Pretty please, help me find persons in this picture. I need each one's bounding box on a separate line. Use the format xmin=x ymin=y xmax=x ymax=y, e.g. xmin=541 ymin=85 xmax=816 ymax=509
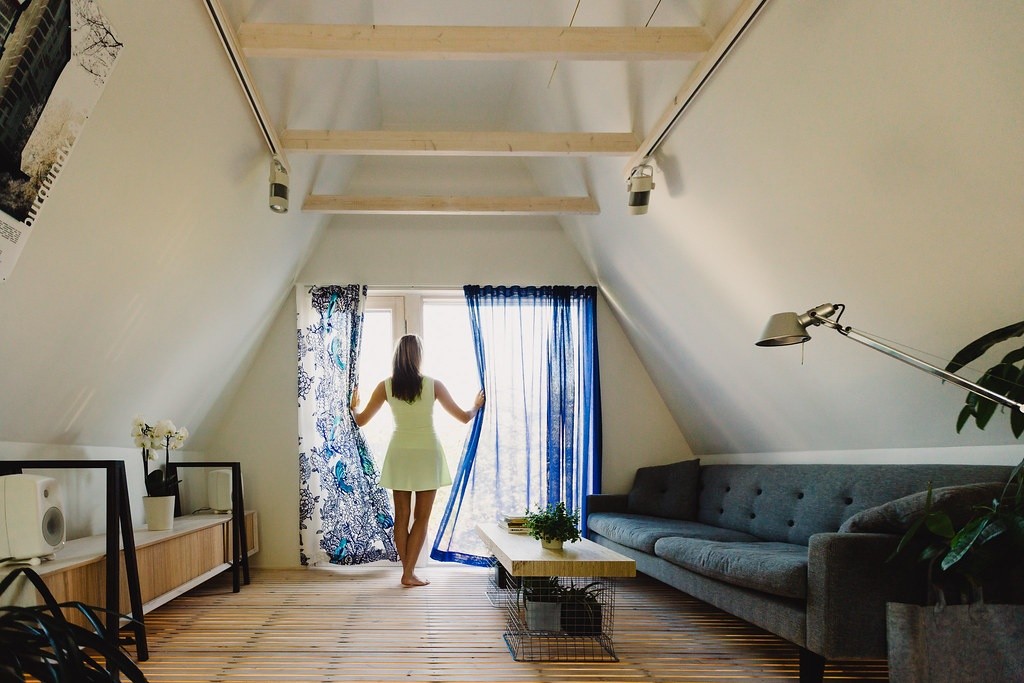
xmin=351 ymin=335 xmax=485 ymax=586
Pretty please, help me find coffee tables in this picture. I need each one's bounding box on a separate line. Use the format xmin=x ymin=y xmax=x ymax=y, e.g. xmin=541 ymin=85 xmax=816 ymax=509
xmin=476 ymin=523 xmax=636 ymax=663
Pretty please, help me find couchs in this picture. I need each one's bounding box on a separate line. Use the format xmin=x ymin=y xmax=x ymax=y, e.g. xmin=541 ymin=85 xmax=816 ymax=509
xmin=584 ymin=463 xmax=1024 ymax=683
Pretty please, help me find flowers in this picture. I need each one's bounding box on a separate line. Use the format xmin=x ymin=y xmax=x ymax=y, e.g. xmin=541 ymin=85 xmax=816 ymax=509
xmin=131 ymin=415 xmax=188 ymax=497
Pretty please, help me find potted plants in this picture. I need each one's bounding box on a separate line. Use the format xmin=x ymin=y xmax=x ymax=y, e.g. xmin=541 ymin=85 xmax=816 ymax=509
xmin=517 ymin=575 xmax=572 ymax=632
xmin=521 ymin=500 xmax=582 ymax=549
xmin=562 ymin=579 xmax=609 ymax=636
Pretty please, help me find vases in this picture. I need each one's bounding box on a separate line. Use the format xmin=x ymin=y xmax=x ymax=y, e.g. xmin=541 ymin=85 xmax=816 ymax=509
xmin=142 ymin=496 xmax=175 ymax=532
xmin=495 ymin=563 xmax=522 ymax=589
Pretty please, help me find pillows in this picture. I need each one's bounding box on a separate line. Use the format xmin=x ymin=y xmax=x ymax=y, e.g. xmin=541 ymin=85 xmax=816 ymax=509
xmin=624 ymin=458 xmax=701 ymax=522
xmin=836 ymin=482 xmax=1018 ymax=534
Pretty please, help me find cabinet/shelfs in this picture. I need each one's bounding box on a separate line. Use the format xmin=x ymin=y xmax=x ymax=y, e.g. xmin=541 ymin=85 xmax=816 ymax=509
xmin=0 ymin=460 xmax=260 ymax=664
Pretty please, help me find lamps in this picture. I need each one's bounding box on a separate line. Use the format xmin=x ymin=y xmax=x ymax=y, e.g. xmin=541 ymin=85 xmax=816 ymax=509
xmin=269 ymin=154 xmax=289 ymax=213
xmin=627 ymin=165 xmax=655 ymax=215
xmin=755 ymin=303 xmax=1024 ymax=414
